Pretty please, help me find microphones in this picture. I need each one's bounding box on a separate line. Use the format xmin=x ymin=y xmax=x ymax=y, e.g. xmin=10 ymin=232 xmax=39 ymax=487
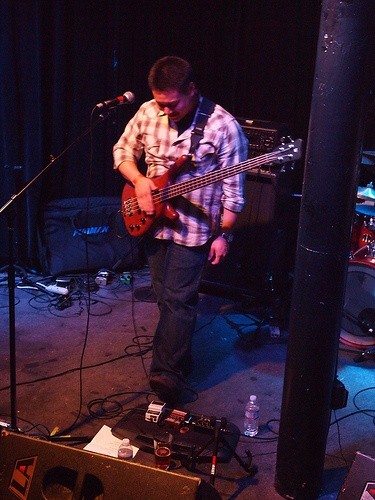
xmin=96 ymin=91 xmax=134 ymax=109
xmin=360 ymin=320 xmax=375 ymax=333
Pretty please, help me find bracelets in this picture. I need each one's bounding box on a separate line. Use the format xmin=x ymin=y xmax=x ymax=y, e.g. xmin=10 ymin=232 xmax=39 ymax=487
xmin=133 ymin=174 xmax=143 ymax=186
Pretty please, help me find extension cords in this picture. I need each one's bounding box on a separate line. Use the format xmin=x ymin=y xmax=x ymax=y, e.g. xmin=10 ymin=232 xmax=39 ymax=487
xmin=46 ymin=284 xmax=69 ymax=295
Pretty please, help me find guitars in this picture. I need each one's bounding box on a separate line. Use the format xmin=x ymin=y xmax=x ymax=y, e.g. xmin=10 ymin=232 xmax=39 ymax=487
xmin=120 ymin=131 xmax=303 ymax=236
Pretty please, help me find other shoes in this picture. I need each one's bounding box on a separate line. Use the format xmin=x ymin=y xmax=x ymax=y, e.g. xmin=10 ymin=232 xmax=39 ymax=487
xmin=149 ymin=373 xmax=182 ymax=403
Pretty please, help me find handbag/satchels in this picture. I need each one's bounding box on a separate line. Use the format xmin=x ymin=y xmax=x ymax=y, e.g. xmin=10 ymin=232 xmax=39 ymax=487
xmin=34 ymin=195 xmax=145 ymax=279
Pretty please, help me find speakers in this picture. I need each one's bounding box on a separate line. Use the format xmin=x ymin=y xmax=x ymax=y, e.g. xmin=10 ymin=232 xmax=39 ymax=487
xmin=197 ymin=172 xmax=302 ymax=312
xmin=0 ymin=427 xmax=224 ymax=500
xmin=338 ymin=451 xmax=375 ymax=500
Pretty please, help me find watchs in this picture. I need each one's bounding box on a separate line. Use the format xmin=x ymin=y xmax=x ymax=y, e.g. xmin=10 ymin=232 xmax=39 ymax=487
xmin=220 ymin=232 xmax=233 ymax=242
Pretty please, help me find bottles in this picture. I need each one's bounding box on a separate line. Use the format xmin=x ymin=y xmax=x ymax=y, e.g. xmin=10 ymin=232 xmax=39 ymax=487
xmin=243 ymin=395 xmax=261 ymax=437
xmin=117 ymin=438 xmax=134 ymax=461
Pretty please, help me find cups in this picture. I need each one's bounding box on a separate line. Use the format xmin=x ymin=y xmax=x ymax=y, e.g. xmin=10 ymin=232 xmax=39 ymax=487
xmin=153 ymin=432 xmax=174 ymax=471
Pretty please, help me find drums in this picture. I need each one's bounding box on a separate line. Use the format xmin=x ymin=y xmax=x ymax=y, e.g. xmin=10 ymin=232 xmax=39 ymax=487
xmin=350 ymin=203 xmax=375 ymax=257
xmin=338 ymin=250 xmax=375 ymax=353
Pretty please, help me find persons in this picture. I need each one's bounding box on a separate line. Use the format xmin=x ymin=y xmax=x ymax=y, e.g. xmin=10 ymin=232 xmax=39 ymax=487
xmin=113 ymin=56 xmax=248 ymax=400
